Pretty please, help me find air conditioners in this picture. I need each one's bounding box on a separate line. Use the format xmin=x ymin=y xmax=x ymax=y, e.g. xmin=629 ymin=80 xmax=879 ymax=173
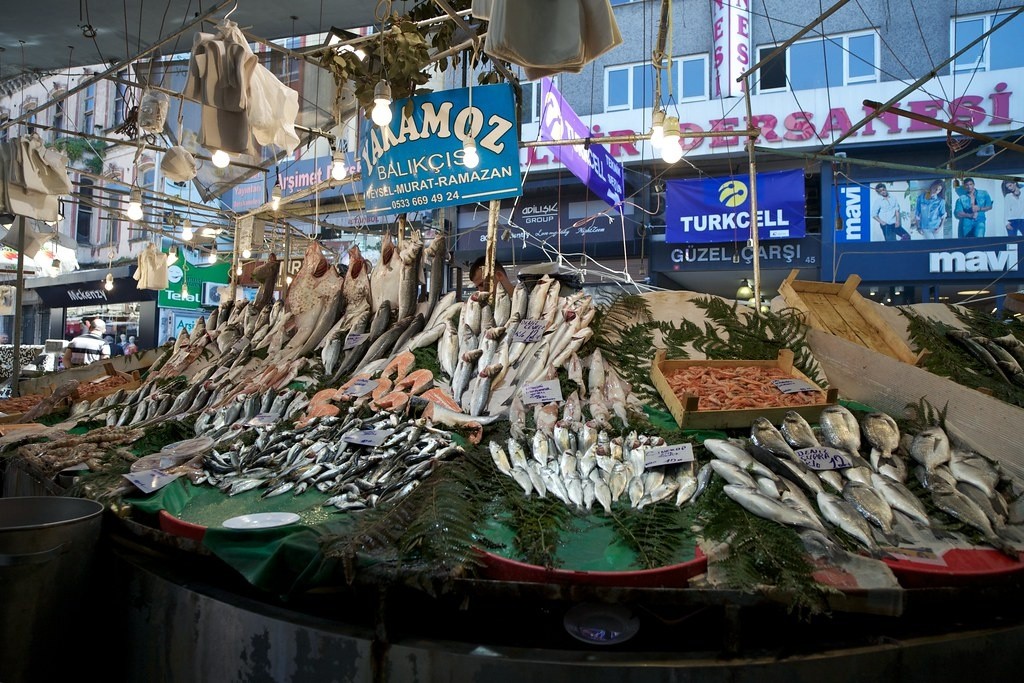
xmin=200 ymin=281 xmax=229 ymax=307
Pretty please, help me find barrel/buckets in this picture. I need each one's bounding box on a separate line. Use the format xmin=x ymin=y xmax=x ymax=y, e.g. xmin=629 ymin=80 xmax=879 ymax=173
xmin=0 ymin=496 xmax=105 ymax=683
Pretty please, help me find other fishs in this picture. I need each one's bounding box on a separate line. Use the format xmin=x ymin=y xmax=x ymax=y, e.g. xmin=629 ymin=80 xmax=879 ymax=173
xmin=490 ymin=418 xmax=712 ymax=516
xmin=992 ymin=331 xmax=1024 ymax=367
xmin=67 ymin=231 xmax=448 ymax=416
xmin=191 ymin=415 xmax=463 ymax=511
xmin=945 ymin=329 xmax=1012 ymax=387
xmin=703 ymin=404 xmax=1024 ymax=561
xmin=973 ymin=336 xmax=1024 ymax=386
xmin=437 ymin=273 xmax=596 ymax=426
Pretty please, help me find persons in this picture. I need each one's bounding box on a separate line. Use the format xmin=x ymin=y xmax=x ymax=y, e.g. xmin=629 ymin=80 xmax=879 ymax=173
xmin=954 ymin=178 xmax=992 ymax=238
xmin=915 ymin=180 xmax=947 ymax=239
xmin=63 ymin=319 xmax=110 ymax=368
xmin=0 ymin=333 xmax=8 ymax=344
xmin=1003 ymin=181 xmax=1024 ymax=236
xmin=469 ymin=256 xmax=514 ymax=299
xmin=125 ymin=336 xmax=138 ymax=355
xmin=872 ymin=184 xmax=911 ymax=240
xmin=104 ymin=336 xmax=124 ymax=357
xmin=118 ymin=334 xmax=127 ymax=350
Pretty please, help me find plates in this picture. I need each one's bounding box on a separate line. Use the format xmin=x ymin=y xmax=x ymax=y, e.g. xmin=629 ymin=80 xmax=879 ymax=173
xmin=221 ymin=512 xmax=301 ymax=529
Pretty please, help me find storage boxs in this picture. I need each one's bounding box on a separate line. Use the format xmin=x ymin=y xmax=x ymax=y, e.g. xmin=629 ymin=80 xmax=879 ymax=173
xmin=651 ymin=350 xmax=839 ymax=427
xmin=777 ymin=269 xmax=934 ymax=369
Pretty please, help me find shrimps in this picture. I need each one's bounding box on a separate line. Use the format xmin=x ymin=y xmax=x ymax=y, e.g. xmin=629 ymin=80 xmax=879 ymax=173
xmin=19 ymin=422 xmax=144 ymax=475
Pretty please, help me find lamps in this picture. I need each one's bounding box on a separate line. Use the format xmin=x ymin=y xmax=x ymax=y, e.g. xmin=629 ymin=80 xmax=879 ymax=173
xmin=735 ymin=278 xmax=754 ymax=301
